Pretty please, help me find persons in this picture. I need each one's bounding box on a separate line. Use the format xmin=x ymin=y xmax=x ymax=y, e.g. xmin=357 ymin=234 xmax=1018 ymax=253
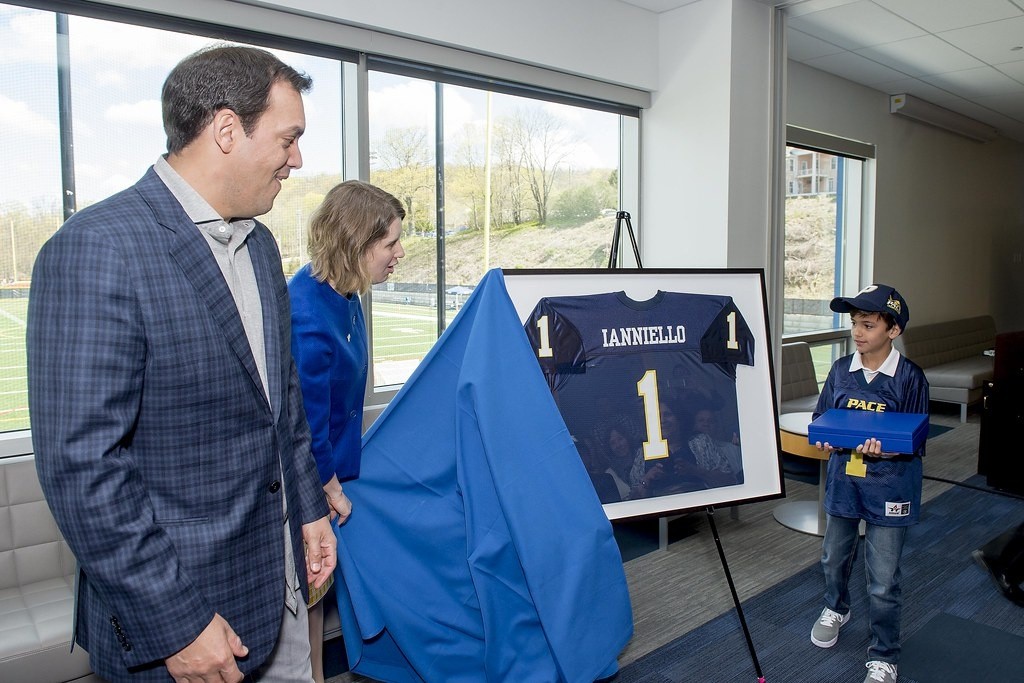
xmin=569 ymin=400 xmax=745 ymax=505
xmin=808 ymin=284 xmax=929 ymax=683
xmin=25 ymin=48 xmax=337 ymax=683
xmin=285 ymin=179 xmax=406 ymax=610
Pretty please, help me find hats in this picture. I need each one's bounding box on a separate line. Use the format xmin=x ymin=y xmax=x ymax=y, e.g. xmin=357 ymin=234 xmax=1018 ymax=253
xmin=830 ymin=284 xmax=909 ymax=336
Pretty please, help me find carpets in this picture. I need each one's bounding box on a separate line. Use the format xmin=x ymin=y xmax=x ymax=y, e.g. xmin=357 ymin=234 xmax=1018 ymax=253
xmin=603 ymin=472 xmax=1024 ymax=683
xmin=927 ymin=423 xmax=955 ymax=439
xmin=611 ymin=520 xmax=700 ymax=565
xmin=781 ymin=452 xmax=820 ymax=486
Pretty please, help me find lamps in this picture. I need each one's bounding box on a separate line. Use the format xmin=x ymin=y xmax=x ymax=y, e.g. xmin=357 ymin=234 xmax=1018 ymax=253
xmin=890 ymin=93 xmax=1000 ymax=143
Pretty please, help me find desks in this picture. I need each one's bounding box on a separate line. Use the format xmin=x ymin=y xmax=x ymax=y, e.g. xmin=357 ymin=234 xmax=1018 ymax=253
xmin=771 ymin=410 xmax=866 ymax=538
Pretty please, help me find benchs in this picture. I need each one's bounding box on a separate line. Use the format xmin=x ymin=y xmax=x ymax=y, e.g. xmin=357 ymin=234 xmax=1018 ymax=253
xmin=891 ymin=313 xmax=998 ymax=424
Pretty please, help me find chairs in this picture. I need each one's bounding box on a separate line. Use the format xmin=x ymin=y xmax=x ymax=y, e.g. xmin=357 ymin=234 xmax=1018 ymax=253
xmin=781 ymin=341 xmax=827 ymax=413
xmin=0 ymin=452 xmax=101 ymax=683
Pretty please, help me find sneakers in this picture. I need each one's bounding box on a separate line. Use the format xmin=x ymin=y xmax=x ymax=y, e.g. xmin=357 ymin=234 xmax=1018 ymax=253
xmin=811 ymin=605 xmax=851 ymax=649
xmin=863 ymin=660 xmax=898 ymax=683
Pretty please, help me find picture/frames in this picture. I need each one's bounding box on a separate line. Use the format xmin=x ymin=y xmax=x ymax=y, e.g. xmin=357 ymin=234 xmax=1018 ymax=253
xmin=499 ymin=266 xmax=788 ymax=522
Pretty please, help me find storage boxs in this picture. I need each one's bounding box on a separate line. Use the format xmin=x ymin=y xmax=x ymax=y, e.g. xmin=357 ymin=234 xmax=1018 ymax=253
xmin=808 ymin=408 xmax=933 ymax=456
